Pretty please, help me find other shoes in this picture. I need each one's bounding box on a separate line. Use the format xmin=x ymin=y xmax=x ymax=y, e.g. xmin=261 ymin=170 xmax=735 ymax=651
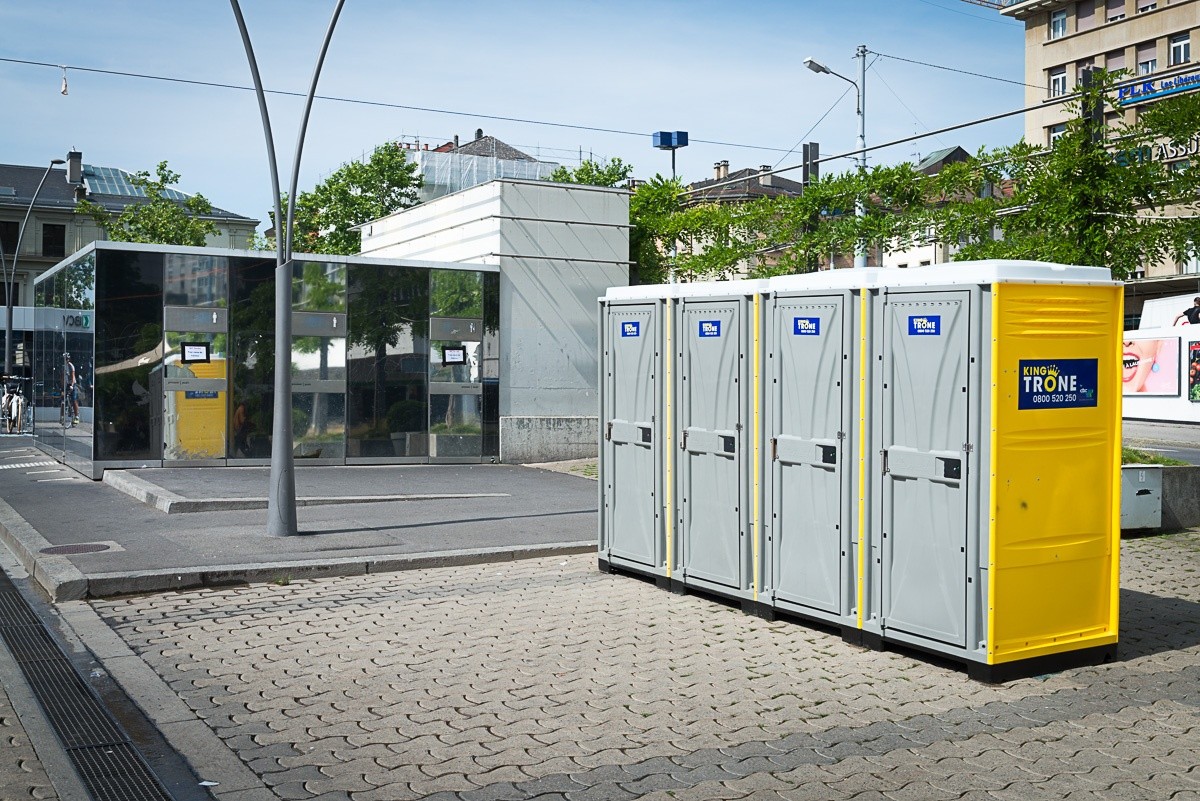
xmin=73 ymin=417 xmax=79 ymax=423
xmin=60 ymin=416 xmax=64 ymax=424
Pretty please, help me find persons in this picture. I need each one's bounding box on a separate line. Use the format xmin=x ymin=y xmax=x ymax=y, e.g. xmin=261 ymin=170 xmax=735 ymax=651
xmin=1122 ymin=339 xmax=1162 ymax=393
xmin=1173 ymin=297 xmax=1200 ymax=326
xmin=235 ymin=399 xmax=251 ymax=457
xmin=57 ymin=352 xmax=80 ymax=423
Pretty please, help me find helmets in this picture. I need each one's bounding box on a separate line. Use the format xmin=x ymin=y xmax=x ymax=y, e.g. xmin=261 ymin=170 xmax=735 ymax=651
xmin=63 ymin=352 xmax=70 ymax=358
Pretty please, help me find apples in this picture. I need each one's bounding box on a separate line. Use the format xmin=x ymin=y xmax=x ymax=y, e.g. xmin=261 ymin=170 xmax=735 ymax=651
xmin=1190 ymin=362 xmax=1200 ymax=384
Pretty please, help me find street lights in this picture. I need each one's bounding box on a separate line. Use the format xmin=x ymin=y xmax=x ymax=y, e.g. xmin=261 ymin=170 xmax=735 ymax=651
xmin=802 ymin=56 xmax=869 ymax=267
xmin=1 ymin=158 xmax=67 ymax=374
xmin=652 ymin=131 xmax=691 ymax=286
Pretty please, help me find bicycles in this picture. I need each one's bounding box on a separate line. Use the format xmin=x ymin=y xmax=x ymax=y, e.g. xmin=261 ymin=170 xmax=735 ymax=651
xmin=60 ymin=388 xmax=79 ymax=429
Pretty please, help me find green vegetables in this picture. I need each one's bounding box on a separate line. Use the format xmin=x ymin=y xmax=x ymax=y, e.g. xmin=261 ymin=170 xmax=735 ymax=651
xmin=1189 ymin=382 xmax=1200 ymax=400
